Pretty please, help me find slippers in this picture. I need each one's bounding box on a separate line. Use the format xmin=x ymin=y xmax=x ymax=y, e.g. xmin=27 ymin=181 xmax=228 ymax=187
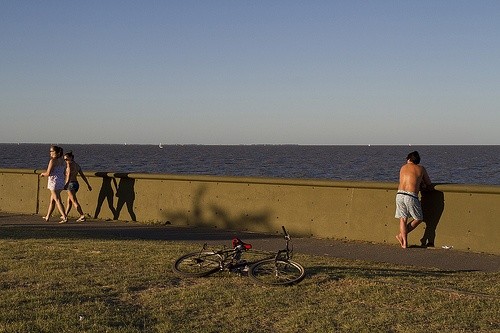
xmin=60 ymin=216 xmax=63 ymax=219
xmin=77 ymin=218 xmax=86 ymax=222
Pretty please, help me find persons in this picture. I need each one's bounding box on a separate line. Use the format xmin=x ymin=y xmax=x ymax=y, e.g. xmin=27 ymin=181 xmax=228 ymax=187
xmin=59 ymin=152 xmax=92 ymax=223
xmin=394 ymin=151 xmax=432 ymax=250
xmin=38 ymin=146 xmax=68 ymax=224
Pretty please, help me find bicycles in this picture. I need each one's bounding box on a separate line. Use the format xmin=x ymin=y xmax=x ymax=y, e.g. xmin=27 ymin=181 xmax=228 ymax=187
xmin=173 ymin=225 xmax=306 ymax=288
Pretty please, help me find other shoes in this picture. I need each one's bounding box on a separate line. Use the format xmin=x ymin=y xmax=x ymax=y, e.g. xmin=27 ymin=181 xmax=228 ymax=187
xmin=59 ymin=220 xmax=68 ymax=223
xmin=42 ymin=216 xmax=48 ymax=221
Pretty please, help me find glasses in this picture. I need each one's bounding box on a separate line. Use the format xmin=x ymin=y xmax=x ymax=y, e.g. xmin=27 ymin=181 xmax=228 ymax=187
xmin=64 ymin=159 xmax=69 ymax=161
